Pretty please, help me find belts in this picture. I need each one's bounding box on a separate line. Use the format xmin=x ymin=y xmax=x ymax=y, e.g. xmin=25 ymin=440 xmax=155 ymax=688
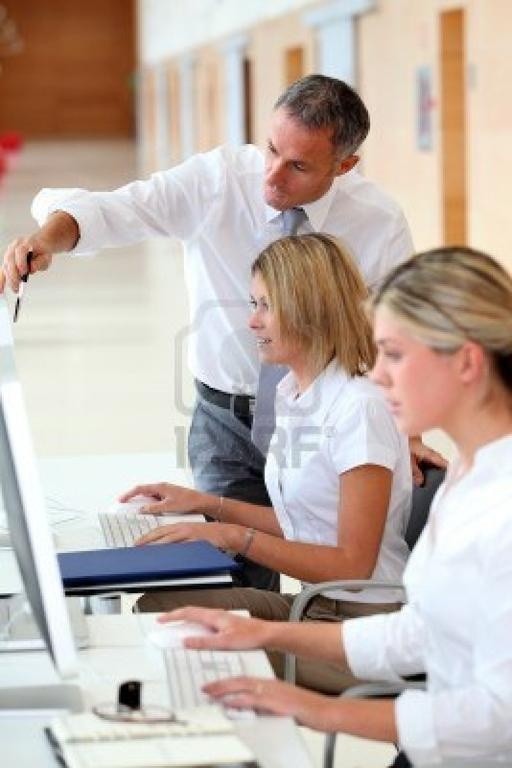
xmin=194 ymin=378 xmax=256 ymax=416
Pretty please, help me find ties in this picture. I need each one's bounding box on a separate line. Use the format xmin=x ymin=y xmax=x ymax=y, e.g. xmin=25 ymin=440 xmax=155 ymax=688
xmin=282 ymin=207 xmax=305 ymax=235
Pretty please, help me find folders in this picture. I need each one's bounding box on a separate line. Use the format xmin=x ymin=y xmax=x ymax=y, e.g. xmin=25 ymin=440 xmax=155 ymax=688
xmin=57 ymin=541 xmax=239 ymax=594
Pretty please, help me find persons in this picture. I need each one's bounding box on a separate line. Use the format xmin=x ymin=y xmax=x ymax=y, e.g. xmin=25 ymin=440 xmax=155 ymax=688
xmin=116 ymin=231 xmax=409 ymax=694
xmin=0 ymin=73 xmax=451 ymax=596
xmin=157 ymin=247 xmax=512 ymax=767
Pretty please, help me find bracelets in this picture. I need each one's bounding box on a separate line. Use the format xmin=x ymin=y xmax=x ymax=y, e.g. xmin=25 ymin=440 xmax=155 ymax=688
xmin=214 ymin=495 xmax=223 ymax=522
xmin=241 ymin=529 xmax=256 ymax=558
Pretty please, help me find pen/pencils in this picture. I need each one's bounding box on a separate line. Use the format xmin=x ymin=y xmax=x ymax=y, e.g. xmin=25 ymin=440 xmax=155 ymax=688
xmin=13 ymin=245 xmax=34 ymax=322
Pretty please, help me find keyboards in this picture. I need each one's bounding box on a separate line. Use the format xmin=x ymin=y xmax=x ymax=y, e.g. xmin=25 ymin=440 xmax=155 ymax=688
xmin=99 ymin=508 xmax=160 ymax=548
xmin=162 ymin=643 xmax=256 ymax=722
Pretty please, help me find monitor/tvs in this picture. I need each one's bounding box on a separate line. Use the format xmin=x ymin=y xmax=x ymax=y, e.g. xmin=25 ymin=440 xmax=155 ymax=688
xmin=0 ymin=297 xmax=94 ymax=680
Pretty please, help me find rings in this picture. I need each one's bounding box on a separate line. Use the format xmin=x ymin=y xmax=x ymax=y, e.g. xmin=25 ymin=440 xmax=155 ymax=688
xmin=258 ymin=684 xmax=266 ymax=695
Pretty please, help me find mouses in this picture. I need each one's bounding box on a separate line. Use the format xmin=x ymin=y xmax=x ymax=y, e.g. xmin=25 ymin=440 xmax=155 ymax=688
xmin=148 ymin=622 xmax=215 ymax=647
xmin=116 ymin=495 xmax=159 ymax=513
